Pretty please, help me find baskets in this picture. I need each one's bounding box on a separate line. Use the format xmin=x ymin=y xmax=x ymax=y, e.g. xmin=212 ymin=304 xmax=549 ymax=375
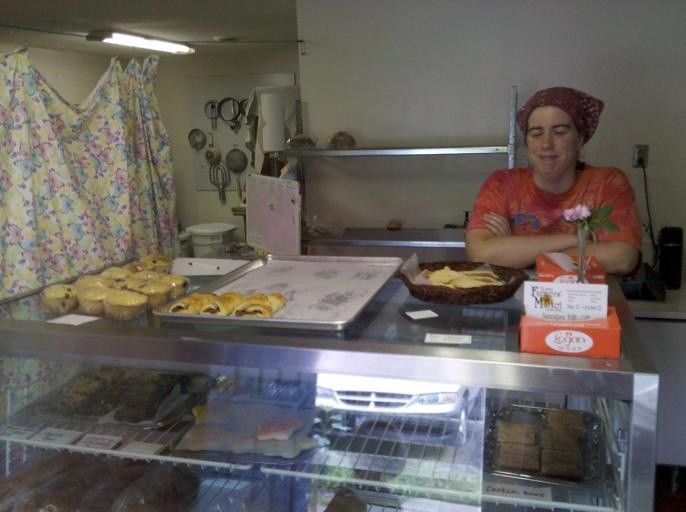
xmin=397 ymin=261 xmax=530 ymax=305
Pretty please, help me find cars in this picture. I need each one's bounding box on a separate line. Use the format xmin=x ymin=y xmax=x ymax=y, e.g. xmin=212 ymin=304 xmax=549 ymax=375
xmin=312 ymin=376 xmax=483 ymax=446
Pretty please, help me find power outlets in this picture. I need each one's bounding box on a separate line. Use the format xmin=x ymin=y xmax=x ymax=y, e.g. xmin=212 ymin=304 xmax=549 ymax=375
xmin=632 ymin=145 xmax=649 ymax=168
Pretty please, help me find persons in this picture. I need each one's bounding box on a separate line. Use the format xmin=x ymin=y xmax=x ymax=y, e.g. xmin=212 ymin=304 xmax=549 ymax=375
xmin=465 ymin=88 xmax=643 ymax=278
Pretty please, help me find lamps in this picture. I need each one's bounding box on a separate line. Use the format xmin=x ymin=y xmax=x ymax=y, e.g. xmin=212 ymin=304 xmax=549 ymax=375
xmin=87 ymin=29 xmax=197 ymax=54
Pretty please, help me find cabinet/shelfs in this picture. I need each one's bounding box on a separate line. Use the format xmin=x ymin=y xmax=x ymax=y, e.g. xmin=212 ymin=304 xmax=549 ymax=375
xmin=267 ymin=84 xmax=519 ymax=255
xmin=0 ymin=253 xmax=660 ymax=512
xmin=633 ymin=320 xmax=685 ymax=496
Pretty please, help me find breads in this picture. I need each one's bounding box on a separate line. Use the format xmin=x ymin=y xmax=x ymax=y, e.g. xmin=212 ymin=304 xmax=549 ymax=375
xmin=418 ymin=264 xmax=505 ymax=288
xmin=40 ymin=254 xmax=287 ymax=321
xmin=495 ymin=410 xmax=586 ymax=480
xmin=0 ymin=371 xmax=366 ymax=512
